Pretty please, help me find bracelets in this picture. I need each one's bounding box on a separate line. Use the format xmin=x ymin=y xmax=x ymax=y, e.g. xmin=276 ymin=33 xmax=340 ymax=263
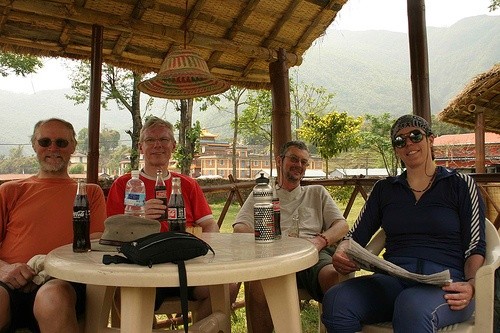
xmin=315 ymin=233 xmax=328 ymax=248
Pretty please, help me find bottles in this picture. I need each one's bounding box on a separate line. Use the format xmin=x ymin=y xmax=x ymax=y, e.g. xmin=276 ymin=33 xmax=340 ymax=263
xmin=155 ymin=169 xmax=168 ymax=221
xmin=287 ymin=214 xmax=300 ymax=240
xmin=72 ymin=179 xmax=91 ymax=253
xmin=252 ymin=172 xmax=275 ymax=243
xmin=123 ymin=170 xmax=147 ymax=219
xmin=271 ymin=197 xmax=282 ymax=240
xmin=166 ymin=177 xmax=187 ymax=234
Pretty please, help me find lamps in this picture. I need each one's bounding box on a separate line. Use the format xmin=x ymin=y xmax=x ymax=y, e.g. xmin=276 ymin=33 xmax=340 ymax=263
xmin=137 ymin=0 xmax=230 ymax=100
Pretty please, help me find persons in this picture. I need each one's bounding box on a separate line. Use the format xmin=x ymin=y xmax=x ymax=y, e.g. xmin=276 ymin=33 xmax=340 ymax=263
xmin=227 ymin=142 xmax=351 ymax=333
xmin=0 ymin=119 xmax=107 ymax=332
xmin=319 ymin=115 xmax=486 ymax=333
xmin=107 ymin=118 xmax=236 ymax=330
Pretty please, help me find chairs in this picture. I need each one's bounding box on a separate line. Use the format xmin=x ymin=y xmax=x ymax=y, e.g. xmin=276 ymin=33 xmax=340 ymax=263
xmin=355 ymin=213 xmax=500 ymax=333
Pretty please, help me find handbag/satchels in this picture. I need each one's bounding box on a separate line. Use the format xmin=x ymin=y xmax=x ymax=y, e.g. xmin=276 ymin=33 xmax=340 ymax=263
xmin=120 ymin=230 xmax=208 ymax=267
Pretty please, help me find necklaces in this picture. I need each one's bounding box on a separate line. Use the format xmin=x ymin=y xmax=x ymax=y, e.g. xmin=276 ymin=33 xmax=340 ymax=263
xmin=405 ymin=166 xmax=437 ymax=193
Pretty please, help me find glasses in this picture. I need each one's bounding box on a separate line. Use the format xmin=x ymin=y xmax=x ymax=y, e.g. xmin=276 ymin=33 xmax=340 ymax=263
xmin=282 ymin=155 xmax=307 ymax=167
xmin=393 ymin=130 xmax=426 ymax=148
xmin=35 ymin=136 xmax=73 ymax=148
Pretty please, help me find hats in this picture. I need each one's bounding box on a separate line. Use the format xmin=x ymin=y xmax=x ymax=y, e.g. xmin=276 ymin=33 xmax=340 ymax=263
xmin=91 ymin=214 xmax=161 ymax=252
xmin=391 ymin=115 xmax=431 ymax=138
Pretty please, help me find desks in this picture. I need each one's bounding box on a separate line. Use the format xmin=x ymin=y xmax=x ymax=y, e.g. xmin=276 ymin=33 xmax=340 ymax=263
xmin=44 ymin=232 xmax=319 ymax=333
